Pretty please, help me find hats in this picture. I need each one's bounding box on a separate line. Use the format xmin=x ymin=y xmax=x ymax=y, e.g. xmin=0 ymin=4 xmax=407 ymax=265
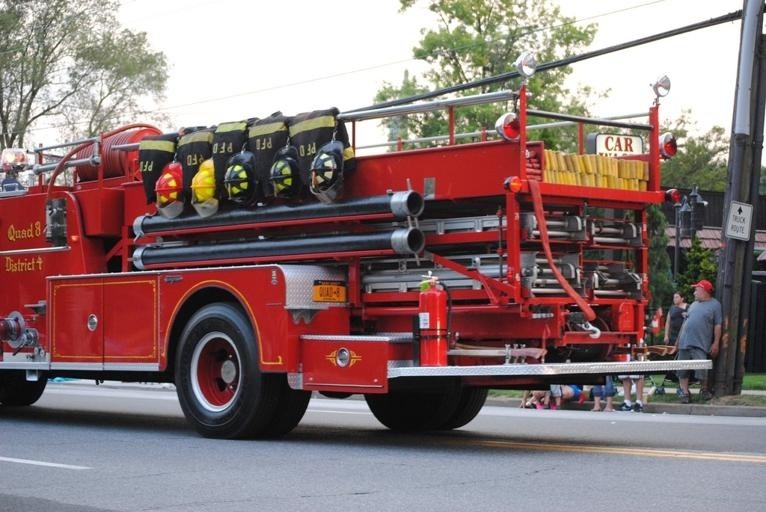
xmin=691 ymin=280 xmax=713 ymax=293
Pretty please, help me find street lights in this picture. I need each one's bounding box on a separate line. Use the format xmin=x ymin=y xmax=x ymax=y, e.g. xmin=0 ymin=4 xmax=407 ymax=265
xmin=673 ymin=182 xmax=708 ymax=280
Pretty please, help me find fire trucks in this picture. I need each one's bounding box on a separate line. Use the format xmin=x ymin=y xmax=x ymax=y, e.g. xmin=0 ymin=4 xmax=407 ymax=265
xmin=0 ymin=51 xmax=713 ymax=436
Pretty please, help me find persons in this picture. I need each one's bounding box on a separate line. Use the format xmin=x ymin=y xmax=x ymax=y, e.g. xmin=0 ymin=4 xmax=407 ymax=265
xmin=520 ymin=338 xmax=646 ymax=412
xmin=664 ymin=291 xmax=690 ymax=346
xmin=677 ymin=280 xmax=723 ymax=404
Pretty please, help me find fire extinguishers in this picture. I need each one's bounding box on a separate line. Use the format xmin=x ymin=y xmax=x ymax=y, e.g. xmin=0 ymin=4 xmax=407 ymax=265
xmin=419 ymin=270 xmax=452 ymax=367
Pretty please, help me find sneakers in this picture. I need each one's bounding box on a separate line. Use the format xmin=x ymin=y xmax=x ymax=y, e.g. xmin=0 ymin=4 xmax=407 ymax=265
xmin=680 ymin=392 xmax=690 ymax=403
xmin=591 ymin=402 xmax=644 ymax=412
xmin=520 ymin=400 xmax=563 ymax=410
xmin=701 ymin=391 xmax=712 ymax=400
